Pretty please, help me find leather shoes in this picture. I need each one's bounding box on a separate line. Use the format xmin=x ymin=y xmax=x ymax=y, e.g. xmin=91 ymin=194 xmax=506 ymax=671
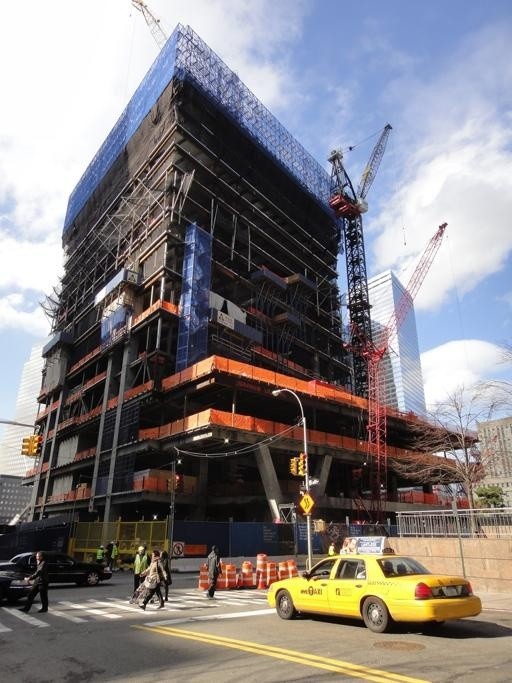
xmin=158 ymin=603 xmax=164 ymax=609
xmin=139 ymin=605 xmax=145 ymax=609
xmin=18 ymin=607 xmax=29 ymax=613
xmin=38 ymin=608 xmax=48 ymax=613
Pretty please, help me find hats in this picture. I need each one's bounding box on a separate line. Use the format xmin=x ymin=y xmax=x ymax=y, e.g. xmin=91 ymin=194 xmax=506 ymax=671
xmin=136 ymin=546 xmax=145 ymax=552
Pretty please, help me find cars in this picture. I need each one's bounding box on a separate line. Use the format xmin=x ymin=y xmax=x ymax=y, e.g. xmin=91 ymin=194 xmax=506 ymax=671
xmin=1 ymin=570 xmax=35 ymax=602
xmin=266 ymin=553 xmax=483 ymax=634
xmin=0 ymin=550 xmax=113 ymax=586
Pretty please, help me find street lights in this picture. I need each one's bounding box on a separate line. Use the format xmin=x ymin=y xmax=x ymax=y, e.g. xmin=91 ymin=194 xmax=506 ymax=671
xmin=271 ymin=387 xmax=313 ymax=569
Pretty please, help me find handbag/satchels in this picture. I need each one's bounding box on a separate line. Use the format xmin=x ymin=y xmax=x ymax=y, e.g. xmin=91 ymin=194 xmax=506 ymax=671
xmin=156 ymin=567 xmax=163 ymax=583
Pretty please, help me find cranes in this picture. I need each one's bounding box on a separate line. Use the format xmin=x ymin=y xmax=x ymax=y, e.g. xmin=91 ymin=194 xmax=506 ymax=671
xmin=326 ymin=122 xmax=393 ymax=399
xmin=342 ymin=221 xmax=449 ymax=502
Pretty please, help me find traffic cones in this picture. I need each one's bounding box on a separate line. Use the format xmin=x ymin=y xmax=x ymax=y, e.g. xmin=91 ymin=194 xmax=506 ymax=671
xmin=215 ymin=553 xmax=298 ymax=591
xmin=197 ymin=563 xmax=210 ymax=592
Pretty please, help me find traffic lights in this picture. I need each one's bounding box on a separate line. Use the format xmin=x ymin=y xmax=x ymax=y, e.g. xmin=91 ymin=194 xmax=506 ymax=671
xmin=21 ymin=436 xmax=32 ymax=456
xmin=289 ymin=457 xmax=297 ymax=476
xmin=300 ymin=452 xmax=307 ymax=470
xmin=31 ymin=436 xmax=42 ymax=456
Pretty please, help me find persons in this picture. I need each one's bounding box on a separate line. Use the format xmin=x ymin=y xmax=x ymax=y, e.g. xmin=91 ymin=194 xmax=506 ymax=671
xmin=207 ymin=545 xmax=222 ymax=598
xmin=328 ymin=543 xmax=336 ymax=556
xmin=18 ymin=552 xmax=48 ymax=612
xmin=139 ymin=552 xmax=167 ymax=611
xmin=155 ymin=551 xmax=172 ymax=601
xmin=134 ymin=545 xmax=151 ymax=591
xmin=96 ymin=539 xmax=119 ymax=572
